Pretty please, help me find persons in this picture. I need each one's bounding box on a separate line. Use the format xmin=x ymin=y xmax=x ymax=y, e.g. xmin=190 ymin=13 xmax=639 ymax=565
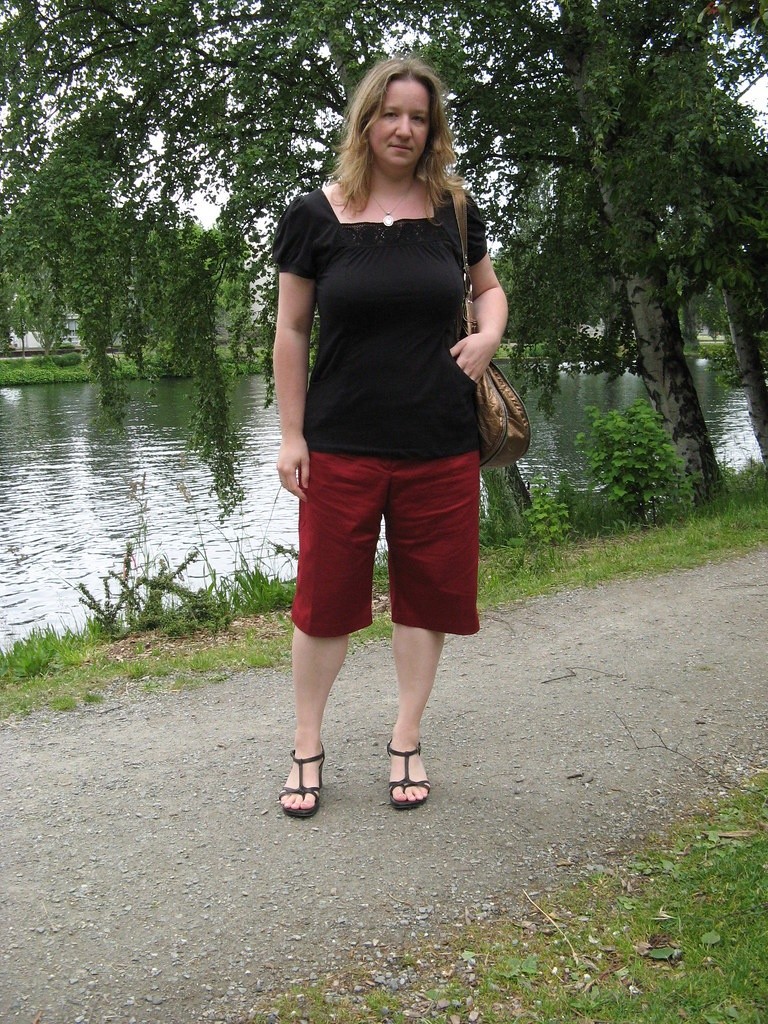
xmin=273 ymin=62 xmax=508 ymax=817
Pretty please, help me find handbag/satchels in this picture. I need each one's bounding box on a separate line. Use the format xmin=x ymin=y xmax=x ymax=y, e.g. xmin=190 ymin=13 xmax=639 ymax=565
xmin=451 ymin=184 xmax=530 ymax=466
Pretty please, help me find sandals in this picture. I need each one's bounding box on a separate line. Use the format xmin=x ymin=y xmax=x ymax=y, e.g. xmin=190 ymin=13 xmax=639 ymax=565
xmin=280 ymin=742 xmax=326 ymax=819
xmin=385 ymin=739 xmax=431 ymax=811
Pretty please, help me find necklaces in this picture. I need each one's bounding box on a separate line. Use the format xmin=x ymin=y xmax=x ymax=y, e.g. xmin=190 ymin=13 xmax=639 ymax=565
xmin=370 ymin=183 xmax=412 ymax=227
